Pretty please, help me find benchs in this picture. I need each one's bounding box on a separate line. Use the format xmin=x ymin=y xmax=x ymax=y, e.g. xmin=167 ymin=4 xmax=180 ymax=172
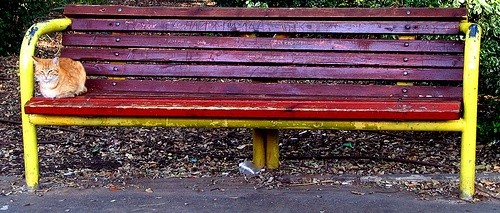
xmin=19 ymin=4 xmax=482 ymax=201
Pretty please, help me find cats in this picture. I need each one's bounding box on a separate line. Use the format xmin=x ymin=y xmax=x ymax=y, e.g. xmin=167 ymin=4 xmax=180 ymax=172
xmin=32 ymin=55 xmax=87 ymax=99
